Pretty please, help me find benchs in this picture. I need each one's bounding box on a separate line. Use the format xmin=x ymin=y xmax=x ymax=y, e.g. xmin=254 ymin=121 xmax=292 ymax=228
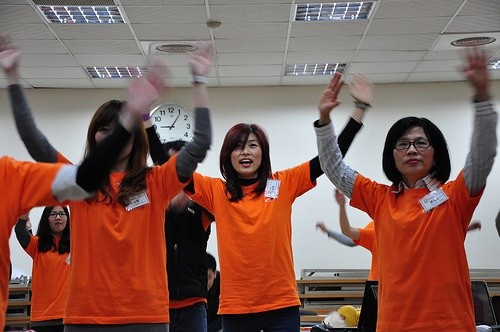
xmin=5 ymin=277 xmax=500 ymax=332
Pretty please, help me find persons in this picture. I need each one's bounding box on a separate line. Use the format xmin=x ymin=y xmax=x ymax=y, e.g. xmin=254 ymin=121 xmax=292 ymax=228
xmin=206 ymin=252 xmax=223 ymax=332
xmin=129 ymin=72 xmax=373 ymax=332
xmin=314 ymin=45 xmax=498 ymax=332
xmin=0 ymin=31 xmax=213 ymax=332
xmin=0 ymin=61 xmax=171 ymax=331
xmin=152 ymin=140 xmax=216 ymax=331
xmin=15 ymin=206 xmax=70 ymax=332
xmin=316 ymin=218 xmax=481 ymax=332
xmin=334 ymin=188 xmax=378 ymax=332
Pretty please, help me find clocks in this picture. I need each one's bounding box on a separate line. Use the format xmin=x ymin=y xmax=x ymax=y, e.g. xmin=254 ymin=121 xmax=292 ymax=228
xmin=149 ymin=103 xmax=195 ymax=144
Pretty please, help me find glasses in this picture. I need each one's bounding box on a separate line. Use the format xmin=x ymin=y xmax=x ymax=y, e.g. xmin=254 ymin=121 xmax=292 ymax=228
xmin=393 ymin=139 xmax=431 ymax=150
xmin=48 ymin=211 xmax=67 ymax=218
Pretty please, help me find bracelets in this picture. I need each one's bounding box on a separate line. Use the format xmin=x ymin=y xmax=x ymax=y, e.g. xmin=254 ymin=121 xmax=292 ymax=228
xmin=353 ymin=100 xmax=368 ymax=110
xmin=191 ymin=74 xmax=209 ymax=84
xmin=142 ymin=113 xmax=151 ymax=122
